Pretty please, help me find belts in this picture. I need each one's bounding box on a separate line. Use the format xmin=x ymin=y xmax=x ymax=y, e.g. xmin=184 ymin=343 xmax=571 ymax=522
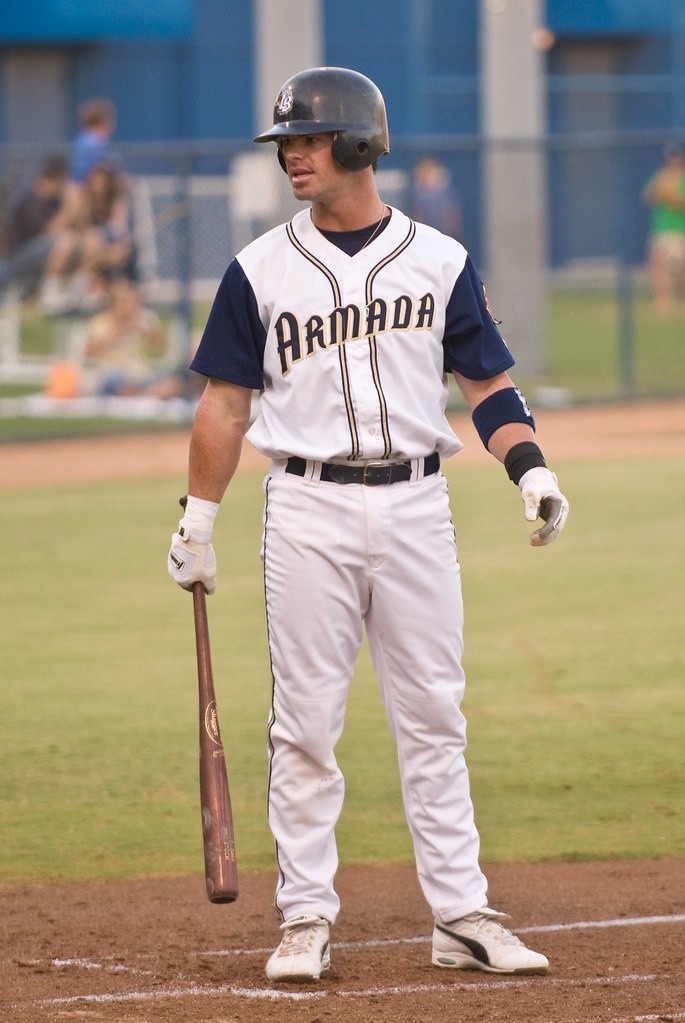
xmin=284 ymin=452 xmax=440 ymax=487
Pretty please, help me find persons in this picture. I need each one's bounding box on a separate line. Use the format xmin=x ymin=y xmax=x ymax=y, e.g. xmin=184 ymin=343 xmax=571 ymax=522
xmin=166 ymin=66 xmax=569 ymax=982
xmin=640 ymin=140 xmax=685 ymax=301
xmin=9 ymin=94 xmax=199 ymax=398
xmin=405 ymin=159 xmax=461 ymax=245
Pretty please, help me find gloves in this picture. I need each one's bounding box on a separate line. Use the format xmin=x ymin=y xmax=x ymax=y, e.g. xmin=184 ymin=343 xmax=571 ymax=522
xmin=167 ymin=495 xmax=220 ymax=595
xmin=517 ymin=466 xmax=568 ymax=546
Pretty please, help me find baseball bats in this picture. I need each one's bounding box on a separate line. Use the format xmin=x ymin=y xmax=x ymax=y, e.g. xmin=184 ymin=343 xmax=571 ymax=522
xmin=178 ymin=494 xmax=241 ymax=907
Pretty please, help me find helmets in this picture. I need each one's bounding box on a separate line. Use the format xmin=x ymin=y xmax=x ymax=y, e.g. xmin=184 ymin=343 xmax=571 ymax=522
xmin=254 ymin=67 xmax=389 ymax=174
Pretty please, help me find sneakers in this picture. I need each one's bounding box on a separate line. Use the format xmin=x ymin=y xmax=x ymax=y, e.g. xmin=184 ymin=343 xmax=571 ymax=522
xmin=430 ymin=907 xmax=550 ymax=973
xmin=264 ymin=915 xmax=330 ymax=982
